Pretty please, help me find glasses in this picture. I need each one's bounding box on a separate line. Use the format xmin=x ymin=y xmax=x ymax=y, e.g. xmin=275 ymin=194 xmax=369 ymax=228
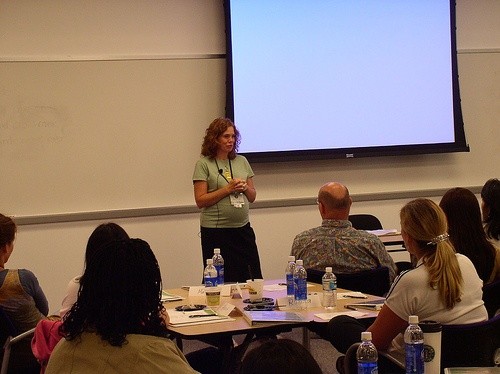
xmin=317 ymin=198 xmax=320 ymax=204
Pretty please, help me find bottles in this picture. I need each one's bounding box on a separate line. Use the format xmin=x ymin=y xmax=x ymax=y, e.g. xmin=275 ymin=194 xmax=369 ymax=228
xmin=405 ymin=315 xmax=424 ymax=374
xmin=203 ymin=259 xmax=218 ymax=286
xmin=294 ymin=260 xmax=307 ymax=310
xmin=212 ymin=248 xmax=224 ymax=290
xmin=322 ymin=266 xmax=337 ymax=311
xmin=356 ymin=331 xmax=378 ymax=374
xmin=287 ymin=255 xmax=296 ymax=306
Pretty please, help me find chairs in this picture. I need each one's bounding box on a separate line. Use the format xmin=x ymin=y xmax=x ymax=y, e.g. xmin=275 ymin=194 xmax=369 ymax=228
xmin=344 ymin=313 xmax=500 ymax=374
xmin=304 ymin=269 xmax=390 ymax=344
xmin=348 ymin=215 xmax=383 ymax=231
xmin=0 ymin=305 xmax=40 ymax=373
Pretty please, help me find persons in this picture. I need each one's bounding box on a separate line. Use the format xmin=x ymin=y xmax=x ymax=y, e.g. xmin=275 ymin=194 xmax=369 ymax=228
xmin=193 ymin=115 xmax=277 ymax=342
xmin=292 ymin=182 xmax=397 ymax=284
xmin=240 ymin=338 xmax=323 ymax=374
xmin=322 ymin=198 xmax=490 ymax=374
xmin=439 ymin=178 xmax=500 ymax=320
xmin=0 ymin=212 xmax=204 ymax=374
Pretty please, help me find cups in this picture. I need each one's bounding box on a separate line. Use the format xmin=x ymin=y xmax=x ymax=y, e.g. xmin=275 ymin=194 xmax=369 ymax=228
xmin=204 ymin=286 xmax=221 ymax=308
xmin=418 ymin=318 xmax=443 ymax=374
xmin=246 ymin=278 xmax=264 ymax=303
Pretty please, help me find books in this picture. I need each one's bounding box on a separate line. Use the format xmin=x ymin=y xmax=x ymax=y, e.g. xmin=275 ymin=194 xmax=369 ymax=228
xmin=344 ymin=298 xmax=386 ymax=311
xmin=365 ymin=229 xmax=402 ymax=236
xmin=241 ymin=311 xmax=303 ymax=326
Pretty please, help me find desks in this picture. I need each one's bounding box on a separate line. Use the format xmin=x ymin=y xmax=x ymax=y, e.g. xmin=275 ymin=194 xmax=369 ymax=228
xmin=378 ymin=230 xmax=417 ymax=268
xmin=160 ymin=278 xmax=385 ymax=374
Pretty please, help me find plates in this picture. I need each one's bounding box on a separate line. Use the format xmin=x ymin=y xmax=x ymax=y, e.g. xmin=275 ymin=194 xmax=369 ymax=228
xmin=244 ymin=298 xmax=274 ymax=304
xmin=245 ymin=304 xmax=275 ymax=311
xmin=175 ymin=304 xmax=206 ymax=311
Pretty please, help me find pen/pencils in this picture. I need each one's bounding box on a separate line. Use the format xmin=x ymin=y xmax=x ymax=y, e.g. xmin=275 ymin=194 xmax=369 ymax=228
xmin=278 ymin=282 xmax=287 ymax=285
xmin=164 ymin=299 xmax=183 ymax=302
xmin=189 ymin=314 xmax=219 ymax=317
xmin=345 ymin=304 xmax=358 ymax=310
xmin=342 ymin=295 xmax=367 ymax=299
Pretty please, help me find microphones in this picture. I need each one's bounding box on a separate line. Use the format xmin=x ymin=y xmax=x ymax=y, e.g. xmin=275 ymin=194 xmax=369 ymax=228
xmin=219 ymin=169 xmax=223 ymax=173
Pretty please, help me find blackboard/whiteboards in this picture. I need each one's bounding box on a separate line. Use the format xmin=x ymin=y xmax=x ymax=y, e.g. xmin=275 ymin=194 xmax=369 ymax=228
xmin=1 ymin=47 xmax=500 ymax=228
